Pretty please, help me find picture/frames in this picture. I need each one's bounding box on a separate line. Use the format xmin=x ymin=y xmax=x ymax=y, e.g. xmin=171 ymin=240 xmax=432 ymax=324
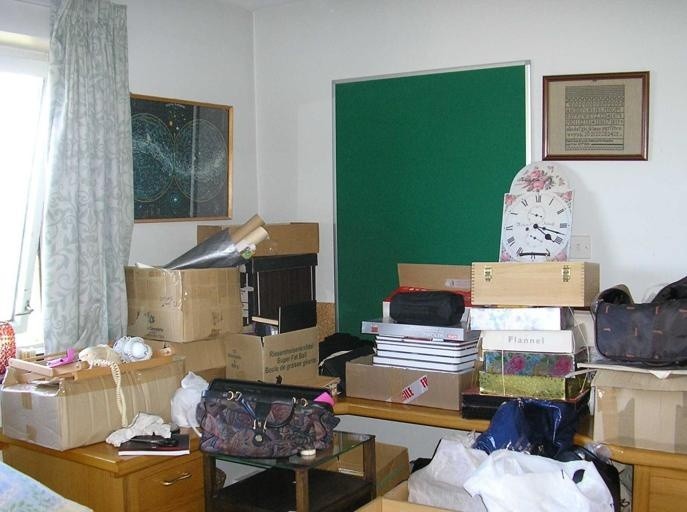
xmin=540 ymin=71 xmax=650 ymax=161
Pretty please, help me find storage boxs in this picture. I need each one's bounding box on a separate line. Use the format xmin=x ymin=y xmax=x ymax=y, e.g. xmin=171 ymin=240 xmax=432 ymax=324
xmin=237 ymin=253 xmax=318 ymax=336
xmin=345 ymin=353 xmax=480 ymax=412
xmin=125 ymin=266 xmax=243 ymax=344
xmin=0 ymin=353 xmax=186 ymax=452
xmin=591 ymin=370 xmax=687 ymax=455
xmin=223 ymin=327 xmax=320 ymax=386
xmin=317 ymin=441 xmax=410 ymax=497
xmin=145 ymin=338 xmax=226 ymax=383
xmin=197 ymin=221 xmax=320 ymax=257
xmin=471 ymin=261 xmax=600 ymax=307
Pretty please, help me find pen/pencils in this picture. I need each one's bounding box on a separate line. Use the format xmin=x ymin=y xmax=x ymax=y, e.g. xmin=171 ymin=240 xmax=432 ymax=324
xmin=129 ymin=438 xmax=177 ymax=449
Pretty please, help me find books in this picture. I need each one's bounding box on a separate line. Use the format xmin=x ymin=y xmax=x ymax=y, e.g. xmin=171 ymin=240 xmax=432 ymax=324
xmin=361 ymin=319 xmax=481 ymax=374
xmin=119 ymin=434 xmax=191 ymax=456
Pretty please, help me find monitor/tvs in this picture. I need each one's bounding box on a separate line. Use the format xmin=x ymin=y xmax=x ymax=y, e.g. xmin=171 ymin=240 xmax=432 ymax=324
xmin=279 ymin=299 xmax=317 ymax=333
xmin=258 ymin=265 xmax=315 ymax=320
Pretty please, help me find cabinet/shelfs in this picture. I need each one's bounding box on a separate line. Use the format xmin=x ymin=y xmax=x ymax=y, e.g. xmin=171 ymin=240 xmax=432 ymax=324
xmin=0 ymin=428 xmax=206 ymax=512
xmin=333 ymin=397 xmax=687 ymax=512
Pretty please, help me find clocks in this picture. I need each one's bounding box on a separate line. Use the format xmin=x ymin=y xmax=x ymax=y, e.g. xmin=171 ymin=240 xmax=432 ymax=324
xmin=499 ymin=162 xmax=575 ymax=263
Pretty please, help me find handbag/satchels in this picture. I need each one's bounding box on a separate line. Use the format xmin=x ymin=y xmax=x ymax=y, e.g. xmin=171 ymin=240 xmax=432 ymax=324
xmin=199 ymin=378 xmax=339 ymax=459
xmin=319 ymin=351 xmax=360 ymax=397
xmin=589 ymin=275 xmax=687 ymax=367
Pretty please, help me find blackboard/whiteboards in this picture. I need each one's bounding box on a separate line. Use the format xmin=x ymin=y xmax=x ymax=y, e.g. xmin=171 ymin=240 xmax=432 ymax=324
xmin=331 ymin=59 xmax=531 ymax=344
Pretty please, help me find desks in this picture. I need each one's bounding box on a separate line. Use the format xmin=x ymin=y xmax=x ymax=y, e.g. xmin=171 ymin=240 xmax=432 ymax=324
xmin=209 ymin=431 xmax=377 ymax=512
